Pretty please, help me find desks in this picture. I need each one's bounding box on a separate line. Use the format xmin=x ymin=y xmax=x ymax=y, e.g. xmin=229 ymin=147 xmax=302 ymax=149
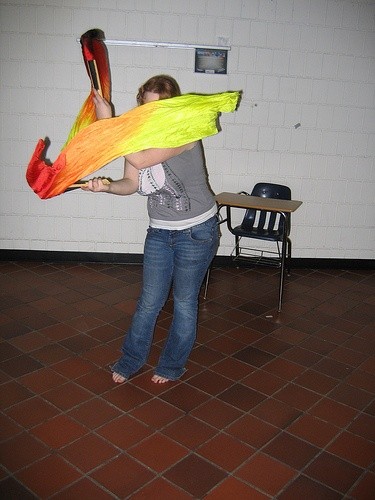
xmin=212 ymin=192 xmax=302 ymax=213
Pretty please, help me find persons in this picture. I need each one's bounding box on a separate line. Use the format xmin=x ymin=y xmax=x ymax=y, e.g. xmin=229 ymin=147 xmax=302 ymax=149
xmin=81 ymin=75 xmax=221 ymax=385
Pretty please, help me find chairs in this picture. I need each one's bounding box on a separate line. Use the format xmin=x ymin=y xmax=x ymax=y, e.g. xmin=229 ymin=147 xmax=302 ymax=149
xmin=204 ymin=183 xmax=303 ymax=312
